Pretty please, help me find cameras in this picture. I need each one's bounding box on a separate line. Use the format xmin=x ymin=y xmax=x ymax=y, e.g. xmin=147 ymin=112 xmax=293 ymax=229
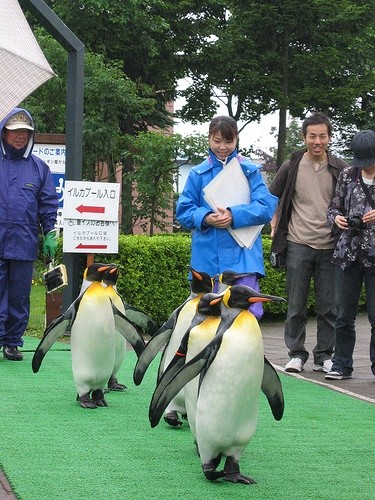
xmin=345 ymin=213 xmax=370 ymax=229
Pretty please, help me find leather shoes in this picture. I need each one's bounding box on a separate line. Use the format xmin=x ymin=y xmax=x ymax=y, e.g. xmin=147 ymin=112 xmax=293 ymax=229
xmin=4 ymin=344 xmax=22 ymax=360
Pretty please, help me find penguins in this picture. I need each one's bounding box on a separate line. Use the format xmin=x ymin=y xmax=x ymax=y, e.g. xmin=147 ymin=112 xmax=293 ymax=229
xmin=149 ymin=285 xmax=288 ymax=485
xmin=149 ymin=292 xmax=225 ymax=458
xmin=133 ymin=266 xmax=215 ymax=428
xmin=216 ymin=270 xmax=255 ymax=295
xmin=32 ymin=263 xmax=146 ymax=409
xmin=43 ymin=265 xmax=158 ymax=389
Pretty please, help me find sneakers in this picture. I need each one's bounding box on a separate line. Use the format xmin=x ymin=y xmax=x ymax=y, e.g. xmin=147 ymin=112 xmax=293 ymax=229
xmin=324 ymin=367 xmax=351 ymax=380
xmin=313 ymin=358 xmax=334 ymax=372
xmin=284 ymin=357 xmax=306 ymax=373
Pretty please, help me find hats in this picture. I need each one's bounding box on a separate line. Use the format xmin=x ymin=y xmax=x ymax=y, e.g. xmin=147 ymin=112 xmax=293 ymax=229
xmin=5 ymin=112 xmax=35 ymax=132
xmin=350 ymin=129 xmax=375 ymax=168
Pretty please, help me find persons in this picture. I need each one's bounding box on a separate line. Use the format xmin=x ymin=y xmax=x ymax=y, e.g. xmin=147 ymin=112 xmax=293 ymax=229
xmin=175 ymin=116 xmax=279 ymax=322
xmin=268 ymin=113 xmax=350 ymax=373
xmin=0 ymin=108 xmax=59 ymax=360
xmin=325 ymin=130 xmax=375 ymax=380
